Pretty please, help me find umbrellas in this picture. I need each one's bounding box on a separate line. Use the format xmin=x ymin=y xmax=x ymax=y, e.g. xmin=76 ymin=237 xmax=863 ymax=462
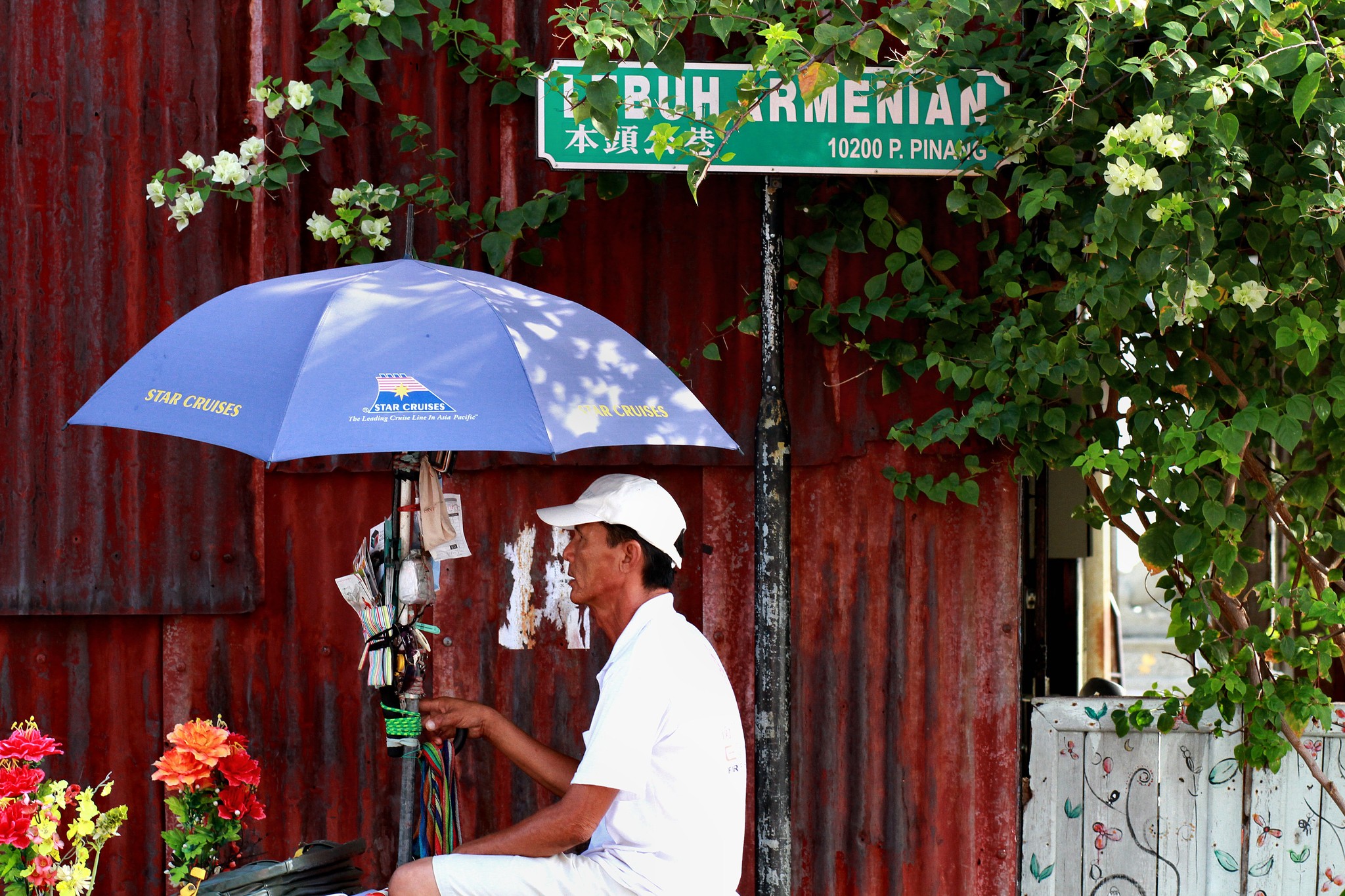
xmin=59 ymin=202 xmax=750 ymax=870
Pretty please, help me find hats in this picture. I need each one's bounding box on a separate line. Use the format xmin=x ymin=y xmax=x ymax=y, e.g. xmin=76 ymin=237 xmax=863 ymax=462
xmin=535 ymin=473 xmax=689 ymax=572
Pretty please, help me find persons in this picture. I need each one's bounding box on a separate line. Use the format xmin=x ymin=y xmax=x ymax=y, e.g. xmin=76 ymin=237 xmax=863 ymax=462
xmin=388 ymin=472 xmax=746 ymax=895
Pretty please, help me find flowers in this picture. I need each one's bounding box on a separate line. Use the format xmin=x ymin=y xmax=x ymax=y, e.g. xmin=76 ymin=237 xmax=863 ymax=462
xmin=144 ymin=711 xmax=272 ymax=896
xmin=0 ymin=711 xmax=132 ymax=896
